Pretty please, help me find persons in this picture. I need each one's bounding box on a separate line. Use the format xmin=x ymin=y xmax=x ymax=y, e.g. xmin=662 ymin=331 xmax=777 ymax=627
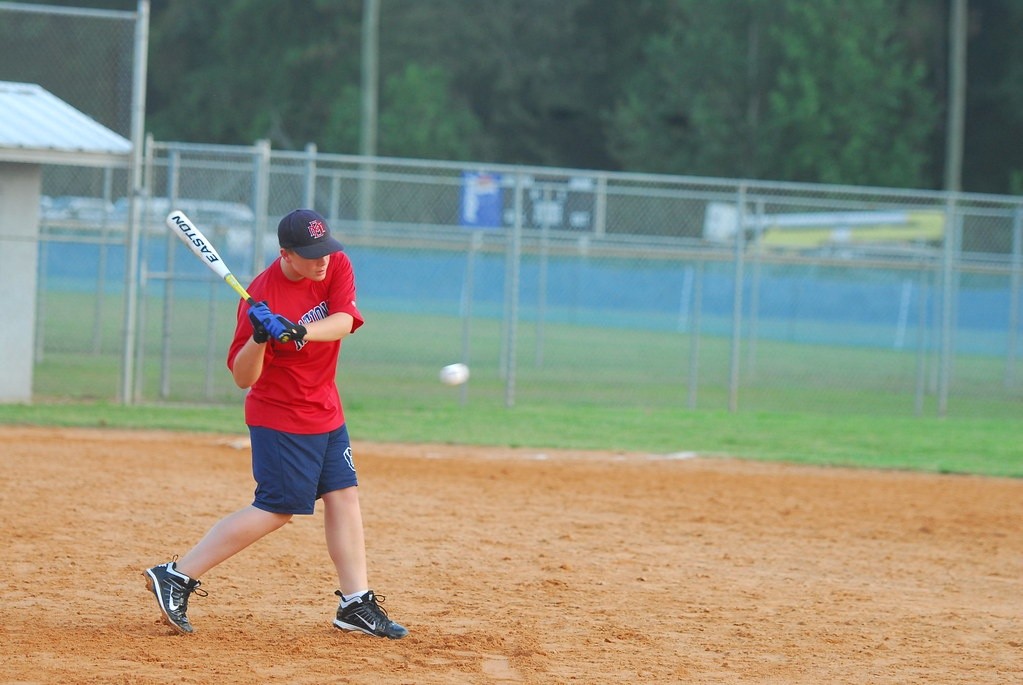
xmin=142 ymin=208 xmax=408 ymax=642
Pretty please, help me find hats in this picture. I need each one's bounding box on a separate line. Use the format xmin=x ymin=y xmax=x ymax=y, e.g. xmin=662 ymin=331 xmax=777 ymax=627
xmin=278 ymin=209 xmax=344 ymax=260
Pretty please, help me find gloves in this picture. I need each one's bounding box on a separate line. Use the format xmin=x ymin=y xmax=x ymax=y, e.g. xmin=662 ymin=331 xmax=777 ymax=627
xmin=246 ymin=300 xmax=273 ymax=344
xmin=262 ymin=314 xmax=307 ymax=343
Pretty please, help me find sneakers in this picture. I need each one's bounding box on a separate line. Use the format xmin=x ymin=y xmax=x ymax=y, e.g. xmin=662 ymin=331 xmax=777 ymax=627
xmin=142 ymin=554 xmax=208 ymax=636
xmin=332 ymin=590 xmax=409 ymax=640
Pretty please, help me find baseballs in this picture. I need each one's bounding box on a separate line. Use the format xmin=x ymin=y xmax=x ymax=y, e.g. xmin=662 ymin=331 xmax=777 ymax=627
xmin=439 ymin=364 xmax=470 ymax=386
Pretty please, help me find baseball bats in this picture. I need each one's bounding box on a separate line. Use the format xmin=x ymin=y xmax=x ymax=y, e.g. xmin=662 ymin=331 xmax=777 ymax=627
xmin=164 ymin=209 xmax=291 ymax=344
